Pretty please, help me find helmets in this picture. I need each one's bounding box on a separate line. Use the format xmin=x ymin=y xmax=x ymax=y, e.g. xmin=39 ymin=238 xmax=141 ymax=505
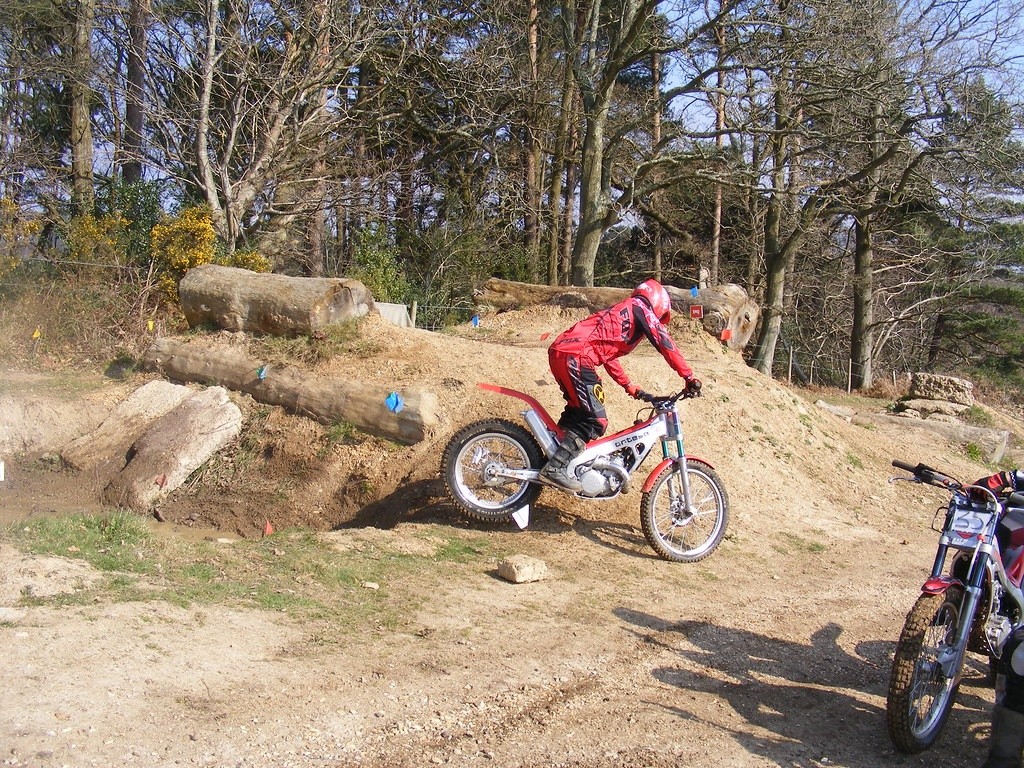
xmin=631 ymin=279 xmax=671 ymax=325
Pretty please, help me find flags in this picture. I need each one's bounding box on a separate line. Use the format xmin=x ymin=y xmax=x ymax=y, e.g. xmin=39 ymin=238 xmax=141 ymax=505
xmin=473 ymin=315 xmax=478 ymax=326
xmin=386 ymin=392 xmax=403 ymax=416
xmin=541 ymin=332 xmax=551 ymax=341
xmin=690 ymin=286 xmax=698 ymax=298
xmin=720 ymin=329 xmax=732 ymax=340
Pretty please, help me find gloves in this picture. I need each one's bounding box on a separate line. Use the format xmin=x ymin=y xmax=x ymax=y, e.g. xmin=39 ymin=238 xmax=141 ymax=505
xmin=684 ymin=374 xmax=702 ymax=393
xmin=969 ymin=471 xmax=1010 ymax=501
xmin=623 ymin=382 xmax=640 ymax=399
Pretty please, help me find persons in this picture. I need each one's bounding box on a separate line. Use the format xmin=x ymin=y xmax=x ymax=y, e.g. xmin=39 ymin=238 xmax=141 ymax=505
xmin=537 ymin=278 xmax=703 ymax=493
xmin=982 ymin=625 xmax=1024 ymax=768
xmin=973 ymin=469 xmax=1024 ymax=497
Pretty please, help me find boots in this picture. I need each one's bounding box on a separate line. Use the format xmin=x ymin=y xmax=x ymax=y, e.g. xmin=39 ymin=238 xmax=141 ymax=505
xmin=538 ymin=431 xmax=586 ymax=493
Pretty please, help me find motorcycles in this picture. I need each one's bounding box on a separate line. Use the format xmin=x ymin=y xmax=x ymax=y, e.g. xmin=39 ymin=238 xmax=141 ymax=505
xmin=882 ymin=457 xmax=1024 ymax=759
xmin=437 ymin=380 xmax=733 ymax=565
